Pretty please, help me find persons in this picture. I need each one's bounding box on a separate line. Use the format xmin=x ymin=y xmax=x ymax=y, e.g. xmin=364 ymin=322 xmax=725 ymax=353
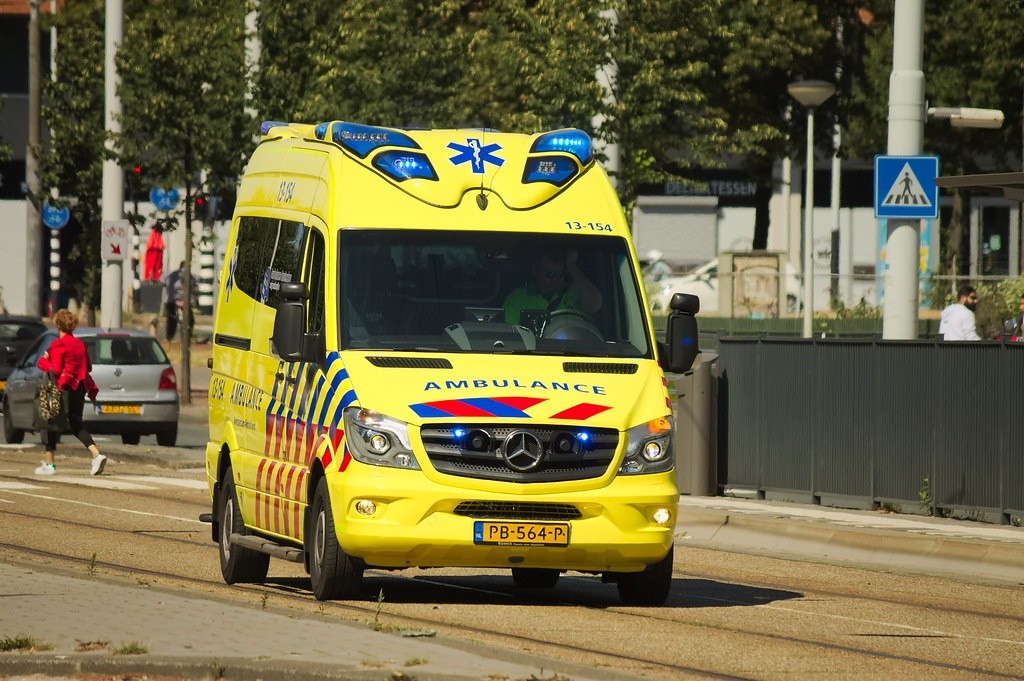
xmin=165 ymin=261 xmax=199 ymax=342
xmin=1019 ymin=293 xmax=1024 ymax=342
xmin=35 ymin=309 xmax=107 ymax=478
xmin=938 ymin=285 xmax=981 ymax=341
xmin=645 ymin=251 xmax=672 ymax=282
xmin=505 ymin=248 xmax=602 ymax=326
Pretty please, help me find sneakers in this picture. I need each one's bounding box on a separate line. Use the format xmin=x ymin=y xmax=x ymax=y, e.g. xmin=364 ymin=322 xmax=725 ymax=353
xmin=91 ymin=455 xmax=107 ymax=475
xmin=35 ymin=462 xmax=56 ymax=475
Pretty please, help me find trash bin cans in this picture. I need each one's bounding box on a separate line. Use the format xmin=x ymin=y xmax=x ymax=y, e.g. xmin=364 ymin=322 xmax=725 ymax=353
xmin=674 ymin=352 xmax=723 ymax=496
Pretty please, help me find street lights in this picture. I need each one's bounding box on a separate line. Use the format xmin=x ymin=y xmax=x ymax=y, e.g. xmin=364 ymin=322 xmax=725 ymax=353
xmin=787 ymin=78 xmax=835 ymax=339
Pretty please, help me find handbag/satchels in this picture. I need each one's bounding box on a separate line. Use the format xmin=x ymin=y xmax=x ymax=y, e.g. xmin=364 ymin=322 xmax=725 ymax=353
xmin=40 ymin=370 xmax=61 ymax=422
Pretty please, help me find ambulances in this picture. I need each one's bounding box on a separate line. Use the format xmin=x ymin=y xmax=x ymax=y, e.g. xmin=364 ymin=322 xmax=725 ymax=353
xmin=198 ymin=120 xmax=704 ymax=602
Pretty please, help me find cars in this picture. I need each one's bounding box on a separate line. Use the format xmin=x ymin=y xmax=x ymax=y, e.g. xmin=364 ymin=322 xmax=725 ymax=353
xmin=0 ymin=313 xmax=49 ymax=412
xmin=2 ymin=326 xmax=180 ymax=446
xmin=645 ymin=252 xmax=806 ymax=319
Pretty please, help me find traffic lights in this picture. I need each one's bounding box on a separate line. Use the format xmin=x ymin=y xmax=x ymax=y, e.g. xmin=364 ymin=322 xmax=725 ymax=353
xmin=131 ymin=163 xmax=143 ymax=177
xmin=194 ymin=195 xmax=208 ymax=221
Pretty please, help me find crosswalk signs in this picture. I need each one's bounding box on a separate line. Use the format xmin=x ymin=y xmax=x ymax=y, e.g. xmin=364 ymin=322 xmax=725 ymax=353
xmin=873 ymin=155 xmax=939 ymax=220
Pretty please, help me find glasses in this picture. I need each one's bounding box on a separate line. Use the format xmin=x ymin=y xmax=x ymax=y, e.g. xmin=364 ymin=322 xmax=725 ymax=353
xmin=547 ymin=272 xmax=569 ymax=278
